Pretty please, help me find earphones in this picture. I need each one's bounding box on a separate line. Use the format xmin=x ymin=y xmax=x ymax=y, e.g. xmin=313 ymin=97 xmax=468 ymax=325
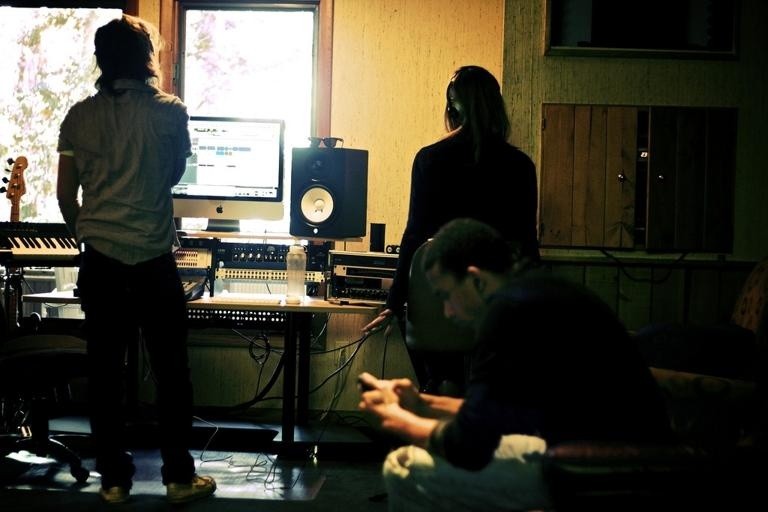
xmin=474 ymin=278 xmax=483 ymax=288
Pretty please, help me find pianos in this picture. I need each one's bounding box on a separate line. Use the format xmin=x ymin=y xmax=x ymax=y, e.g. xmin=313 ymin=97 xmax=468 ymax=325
xmin=0 ymin=220 xmax=80 ymax=265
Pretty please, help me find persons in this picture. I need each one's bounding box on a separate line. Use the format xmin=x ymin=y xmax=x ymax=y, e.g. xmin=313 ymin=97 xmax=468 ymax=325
xmin=360 ymin=217 xmax=671 ymax=509
xmin=56 ymin=15 xmax=217 ymax=501
xmin=360 ymin=66 xmax=541 ymax=395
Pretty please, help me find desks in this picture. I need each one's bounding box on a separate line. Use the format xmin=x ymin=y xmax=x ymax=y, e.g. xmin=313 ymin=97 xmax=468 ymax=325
xmin=20 ymin=283 xmax=385 ymax=473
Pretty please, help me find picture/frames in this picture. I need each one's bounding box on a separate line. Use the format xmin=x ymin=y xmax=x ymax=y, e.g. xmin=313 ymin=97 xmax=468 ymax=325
xmin=543 ymin=2 xmax=741 ymax=62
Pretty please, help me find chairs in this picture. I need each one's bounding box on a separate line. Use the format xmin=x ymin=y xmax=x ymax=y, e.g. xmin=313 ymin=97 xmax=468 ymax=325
xmin=542 ymin=411 xmax=765 ymax=505
xmin=625 ymin=264 xmax=765 ymax=405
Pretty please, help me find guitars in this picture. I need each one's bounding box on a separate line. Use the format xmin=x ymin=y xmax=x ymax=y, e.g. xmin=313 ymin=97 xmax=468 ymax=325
xmin=0 ymin=156 xmax=40 ymax=417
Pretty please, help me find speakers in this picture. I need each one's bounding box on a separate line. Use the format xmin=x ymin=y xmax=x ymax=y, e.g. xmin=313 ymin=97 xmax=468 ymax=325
xmin=291 ymin=148 xmax=368 ymax=238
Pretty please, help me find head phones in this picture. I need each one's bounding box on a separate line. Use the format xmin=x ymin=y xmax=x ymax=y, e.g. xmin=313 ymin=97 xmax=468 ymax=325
xmin=446 ymin=69 xmax=464 ymax=127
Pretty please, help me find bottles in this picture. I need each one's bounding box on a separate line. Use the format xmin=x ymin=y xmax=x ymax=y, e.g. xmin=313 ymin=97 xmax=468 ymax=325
xmin=284 ymin=246 xmax=307 ymax=305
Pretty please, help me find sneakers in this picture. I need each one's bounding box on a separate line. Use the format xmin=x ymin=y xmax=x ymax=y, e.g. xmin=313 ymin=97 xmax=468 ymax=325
xmin=98 ymin=486 xmax=130 ymax=503
xmin=166 ymin=475 xmax=215 ymax=503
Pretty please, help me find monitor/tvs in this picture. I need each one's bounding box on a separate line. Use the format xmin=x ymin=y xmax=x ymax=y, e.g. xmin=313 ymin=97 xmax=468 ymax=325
xmin=170 ymin=115 xmax=285 ymax=202
